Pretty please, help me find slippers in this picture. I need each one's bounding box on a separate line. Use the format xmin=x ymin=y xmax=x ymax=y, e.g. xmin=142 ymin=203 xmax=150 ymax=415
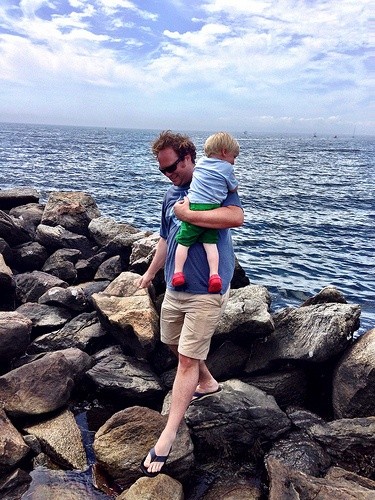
xmin=190 ymin=385 xmax=223 ymax=405
xmin=141 ymin=446 xmax=172 ymax=477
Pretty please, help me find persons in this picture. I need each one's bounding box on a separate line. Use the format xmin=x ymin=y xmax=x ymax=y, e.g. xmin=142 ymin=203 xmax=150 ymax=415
xmin=133 ymin=130 xmax=243 ymax=476
xmin=171 ymin=131 xmax=239 ymax=293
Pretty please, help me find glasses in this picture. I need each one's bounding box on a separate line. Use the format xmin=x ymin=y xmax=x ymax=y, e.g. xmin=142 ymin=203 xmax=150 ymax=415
xmin=159 ymin=158 xmax=180 ymax=174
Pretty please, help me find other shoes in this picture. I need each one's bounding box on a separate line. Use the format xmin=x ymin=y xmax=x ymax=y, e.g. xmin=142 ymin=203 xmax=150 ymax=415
xmin=208 ymin=274 xmax=222 ymax=292
xmin=171 ymin=272 xmax=184 ymax=286
